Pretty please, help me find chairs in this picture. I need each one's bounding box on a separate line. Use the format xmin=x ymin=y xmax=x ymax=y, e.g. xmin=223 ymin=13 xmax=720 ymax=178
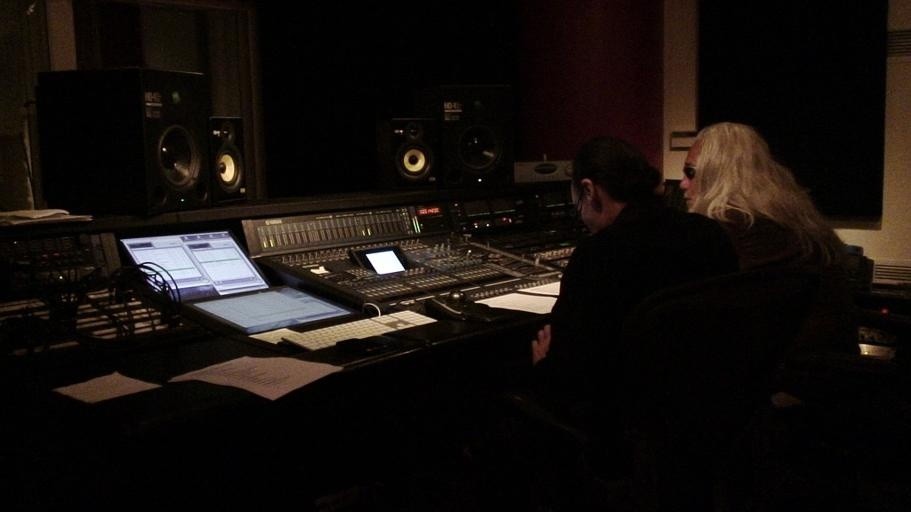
xmin=485 ymin=267 xmax=822 ymax=512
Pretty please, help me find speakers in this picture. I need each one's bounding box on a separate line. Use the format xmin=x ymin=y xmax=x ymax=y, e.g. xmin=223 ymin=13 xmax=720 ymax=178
xmin=391 ymin=118 xmax=441 ymax=188
xmin=436 ymin=83 xmax=509 ymax=186
xmin=37 ymin=68 xmax=211 ymax=214
xmin=210 ymin=117 xmax=248 ymax=207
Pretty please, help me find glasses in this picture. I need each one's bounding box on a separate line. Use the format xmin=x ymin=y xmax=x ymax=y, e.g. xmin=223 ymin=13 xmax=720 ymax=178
xmin=684 ymin=167 xmax=696 ymax=180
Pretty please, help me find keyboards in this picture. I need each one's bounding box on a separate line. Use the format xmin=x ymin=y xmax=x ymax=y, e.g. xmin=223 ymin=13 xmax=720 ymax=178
xmin=280 ymin=310 xmax=437 ymax=349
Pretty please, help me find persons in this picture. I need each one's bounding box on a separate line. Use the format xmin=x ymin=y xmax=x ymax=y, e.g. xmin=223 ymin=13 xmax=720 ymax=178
xmin=528 ymin=134 xmax=740 ymax=511
xmin=678 ymin=121 xmax=861 ymax=511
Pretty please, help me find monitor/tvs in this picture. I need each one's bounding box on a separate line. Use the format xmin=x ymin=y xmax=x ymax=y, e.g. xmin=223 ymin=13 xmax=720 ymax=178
xmin=118 ymin=228 xmax=271 ymax=304
xmin=189 ymin=285 xmax=359 ymax=337
